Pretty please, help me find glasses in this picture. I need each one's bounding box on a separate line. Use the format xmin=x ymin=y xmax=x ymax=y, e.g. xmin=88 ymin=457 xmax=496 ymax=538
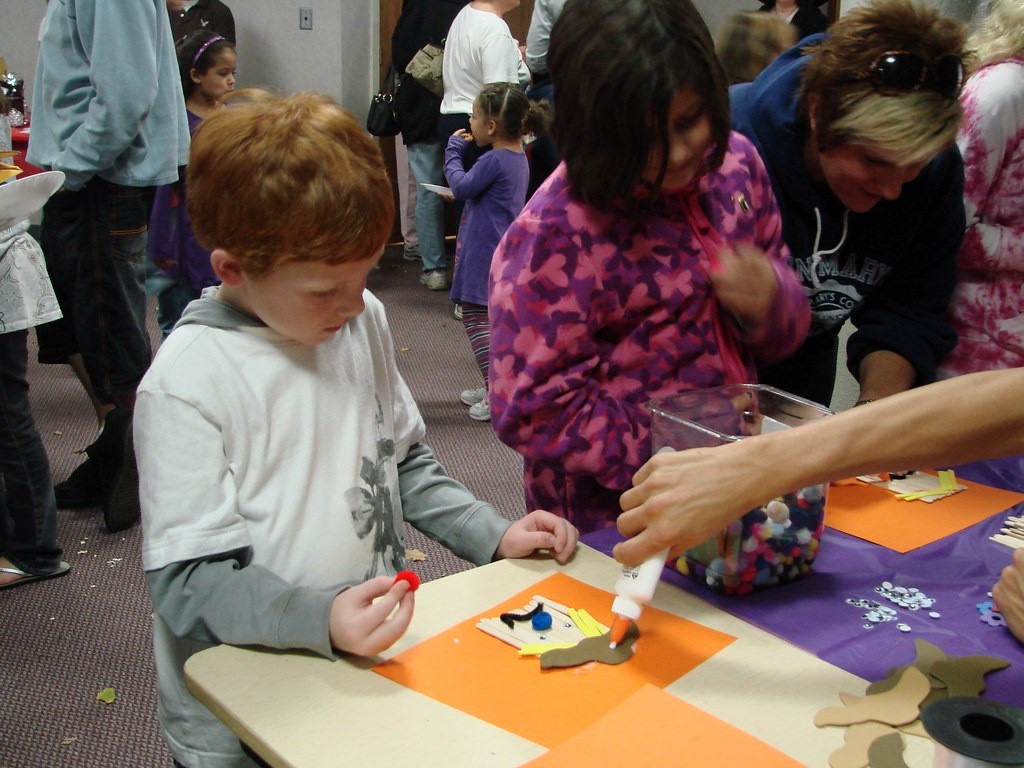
xmin=806 ymin=51 xmax=963 ymax=100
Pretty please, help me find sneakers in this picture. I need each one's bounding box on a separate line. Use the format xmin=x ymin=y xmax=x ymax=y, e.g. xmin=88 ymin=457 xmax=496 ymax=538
xmin=460 ymin=388 xmax=487 ymax=406
xmin=469 ymin=399 xmax=490 ymax=420
xmin=421 ymin=271 xmax=447 ymax=290
xmin=53 ymin=409 xmax=141 ymax=532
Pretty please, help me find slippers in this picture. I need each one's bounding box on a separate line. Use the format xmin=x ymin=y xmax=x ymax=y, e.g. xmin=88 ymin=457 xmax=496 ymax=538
xmin=0 ymin=560 xmax=70 ymax=585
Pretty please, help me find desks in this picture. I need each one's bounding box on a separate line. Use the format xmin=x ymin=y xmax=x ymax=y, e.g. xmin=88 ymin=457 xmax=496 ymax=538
xmin=183 ymin=467 xmax=1024 ymax=768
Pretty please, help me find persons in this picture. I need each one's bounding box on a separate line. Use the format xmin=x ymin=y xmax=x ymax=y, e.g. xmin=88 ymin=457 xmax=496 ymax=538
xmin=132 ymin=89 xmax=580 ymax=768
xmin=390 ymin=0 xmax=1024 ymax=644
xmin=0 ymin=0 xmax=240 ymax=591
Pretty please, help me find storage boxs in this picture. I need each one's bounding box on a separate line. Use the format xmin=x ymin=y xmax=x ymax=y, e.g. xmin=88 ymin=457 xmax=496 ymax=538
xmin=645 ymin=382 xmax=837 ymax=596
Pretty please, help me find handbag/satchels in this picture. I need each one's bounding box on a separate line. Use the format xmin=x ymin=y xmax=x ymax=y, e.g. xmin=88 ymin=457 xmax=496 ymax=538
xmin=367 ymin=63 xmax=400 ymax=136
xmin=405 ymin=43 xmax=445 ymax=97
